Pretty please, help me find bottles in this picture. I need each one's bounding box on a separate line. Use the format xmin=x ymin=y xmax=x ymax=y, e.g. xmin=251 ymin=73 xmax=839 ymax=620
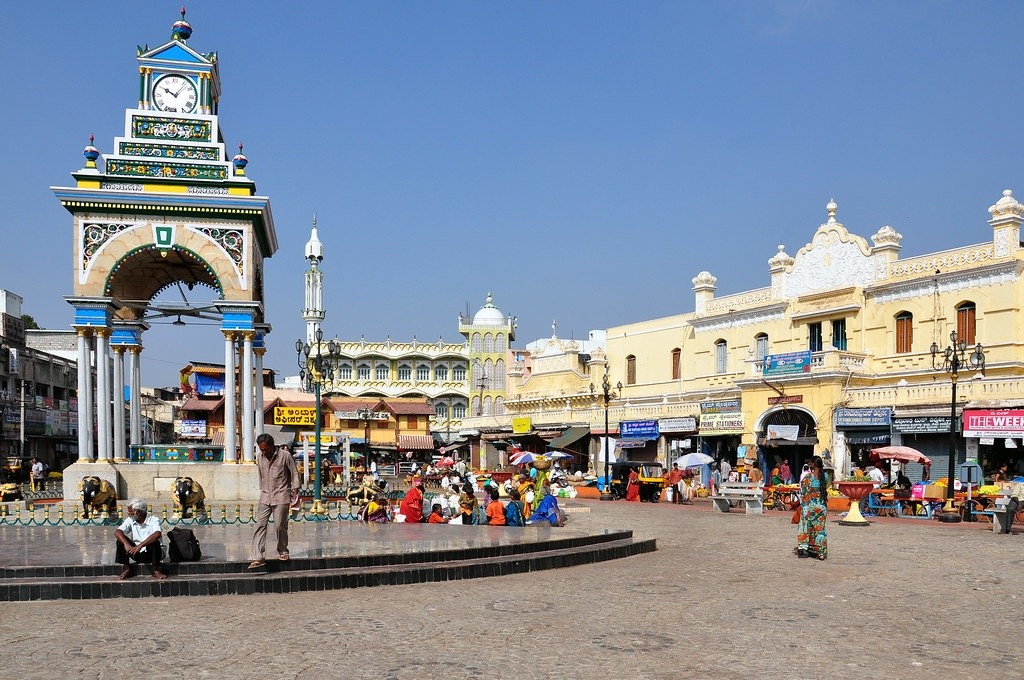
xmin=559 ymin=489 xmax=565 ymax=498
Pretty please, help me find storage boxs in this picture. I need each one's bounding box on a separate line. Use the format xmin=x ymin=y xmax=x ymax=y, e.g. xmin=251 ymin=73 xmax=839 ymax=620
xmin=925 ymin=484 xmax=948 ymax=500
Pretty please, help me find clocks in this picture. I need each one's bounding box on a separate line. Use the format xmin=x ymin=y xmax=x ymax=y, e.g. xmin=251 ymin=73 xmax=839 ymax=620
xmin=154 ymin=77 xmax=197 ymax=114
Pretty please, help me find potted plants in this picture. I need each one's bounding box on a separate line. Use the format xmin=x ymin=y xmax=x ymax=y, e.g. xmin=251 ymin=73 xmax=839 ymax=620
xmin=831 ymin=473 xmax=880 ymax=527
xmin=491 ymin=468 xmax=515 ymax=499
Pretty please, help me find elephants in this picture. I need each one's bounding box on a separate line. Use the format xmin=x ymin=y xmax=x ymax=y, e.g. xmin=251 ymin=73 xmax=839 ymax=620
xmin=77 ymin=475 xmax=118 ymax=520
xmin=170 ymin=476 xmax=208 ymax=519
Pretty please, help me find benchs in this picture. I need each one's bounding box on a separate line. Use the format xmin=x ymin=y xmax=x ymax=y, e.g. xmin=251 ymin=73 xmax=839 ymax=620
xmin=984 ymin=490 xmax=1012 ymax=533
xmin=708 ymin=482 xmax=766 ymax=515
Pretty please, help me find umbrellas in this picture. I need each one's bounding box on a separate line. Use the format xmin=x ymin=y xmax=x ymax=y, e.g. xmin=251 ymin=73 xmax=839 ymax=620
xmin=871 ymin=446 xmax=932 ymax=470
xmin=338 ymin=452 xmax=365 ymax=467
xmin=543 ymin=450 xmax=573 ymax=463
xmin=511 ymin=452 xmax=541 ymax=465
xmin=672 ymin=453 xmax=715 ymax=484
xmin=509 ymin=451 xmax=528 ymax=461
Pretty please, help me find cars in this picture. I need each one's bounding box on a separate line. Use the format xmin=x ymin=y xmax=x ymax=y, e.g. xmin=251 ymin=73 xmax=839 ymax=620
xmin=610 ymin=460 xmax=673 ymax=503
xmin=2 ymin=457 xmax=32 ymax=483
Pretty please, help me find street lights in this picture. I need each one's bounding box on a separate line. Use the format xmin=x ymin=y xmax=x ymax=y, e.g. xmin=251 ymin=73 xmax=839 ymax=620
xmin=356 ymin=405 xmax=374 ymax=475
xmin=930 ymin=331 xmax=984 ymax=523
xmin=589 ymin=374 xmax=622 ymax=501
xmin=145 ymin=397 xmax=158 ymax=443
xmin=294 ymin=328 xmax=340 ymax=521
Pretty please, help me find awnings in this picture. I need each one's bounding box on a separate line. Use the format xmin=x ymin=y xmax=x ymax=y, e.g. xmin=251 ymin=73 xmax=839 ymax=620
xmin=437 ymin=443 xmax=468 ymax=452
xmin=211 ymin=432 xmax=225 ymax=446
xmin=399 ymin=435 xmax=434 ymax=449
xmin=616 ymin=436 xmax=658 ymax=447
xmin=546 ymin=427 xmax=591 ymax=457
xmin=254 ymin=425 xmax=295 ymax=449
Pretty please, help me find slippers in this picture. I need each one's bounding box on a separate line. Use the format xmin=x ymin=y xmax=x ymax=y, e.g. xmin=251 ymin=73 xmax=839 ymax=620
xmin=120 ymin=573 xmax=131 ymax=580
xmin=278 ymin=551 xmax=289 ymax=561
xmin=248 ymin=562 xmax=266 ymax=569
xmin=154 ymin=573 xmax=166 ymax=580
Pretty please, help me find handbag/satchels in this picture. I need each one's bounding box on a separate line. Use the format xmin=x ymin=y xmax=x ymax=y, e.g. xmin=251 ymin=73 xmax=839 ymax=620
xmin=791 ymin=506 xmax=801 ymax=524
xmin=167 ymin=527 xmax=202 ymax=562
xmin=631 ymin=479 xmax=639 ymax=484
xmin=519 ymin=515 xmax=526 ymax=526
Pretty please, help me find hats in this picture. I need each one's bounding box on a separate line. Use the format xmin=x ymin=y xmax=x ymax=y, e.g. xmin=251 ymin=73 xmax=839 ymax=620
xmin=371 ymin=493 xmax=379 ymax=499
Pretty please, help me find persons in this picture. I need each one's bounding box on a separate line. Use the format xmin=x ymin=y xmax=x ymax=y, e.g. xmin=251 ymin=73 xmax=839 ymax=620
xmin=867 ymin=462 xmax=884 ymax=490
xmin=995 ymin=464 xmax=1008 ymax=482
xmin=247 ymin=434 xmax=302 ymax=569
xmin=661 ymin=462 xmax=683 ymax=502
xmin=626 ymin=465 xmax=640 ymax=501
xmin=718 ymin=456 xmax=733 ymax=482
xmin=921 ymin=463 xmax=930 ymax=482
xmin=31 ymin=457 xmax=45 ymax=491
xmin=749 ymin=462 xmax=764 ymax=490
xmin=770 ymin=456 xmax=829 ymax=560
xmin=113 ymin=498 xmax=168 ymax=580
xmin=884 ymin=470 xmax=917 ymax=516
xmin=361 ymin=458 xmax=565 ymax=527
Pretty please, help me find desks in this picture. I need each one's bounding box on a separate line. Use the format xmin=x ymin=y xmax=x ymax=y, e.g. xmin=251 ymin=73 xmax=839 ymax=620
xmin=762 ymin=486 xmax=800 ymax=511
xmin=956 ymin=493 xmax=1024 ymax=525
xmin=880 ymin=496 xmax=941 ymax=520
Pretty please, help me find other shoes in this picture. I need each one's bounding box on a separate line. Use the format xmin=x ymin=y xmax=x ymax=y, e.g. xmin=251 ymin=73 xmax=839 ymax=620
xmin=817 ymin=554 xmax=825 ymax=560
